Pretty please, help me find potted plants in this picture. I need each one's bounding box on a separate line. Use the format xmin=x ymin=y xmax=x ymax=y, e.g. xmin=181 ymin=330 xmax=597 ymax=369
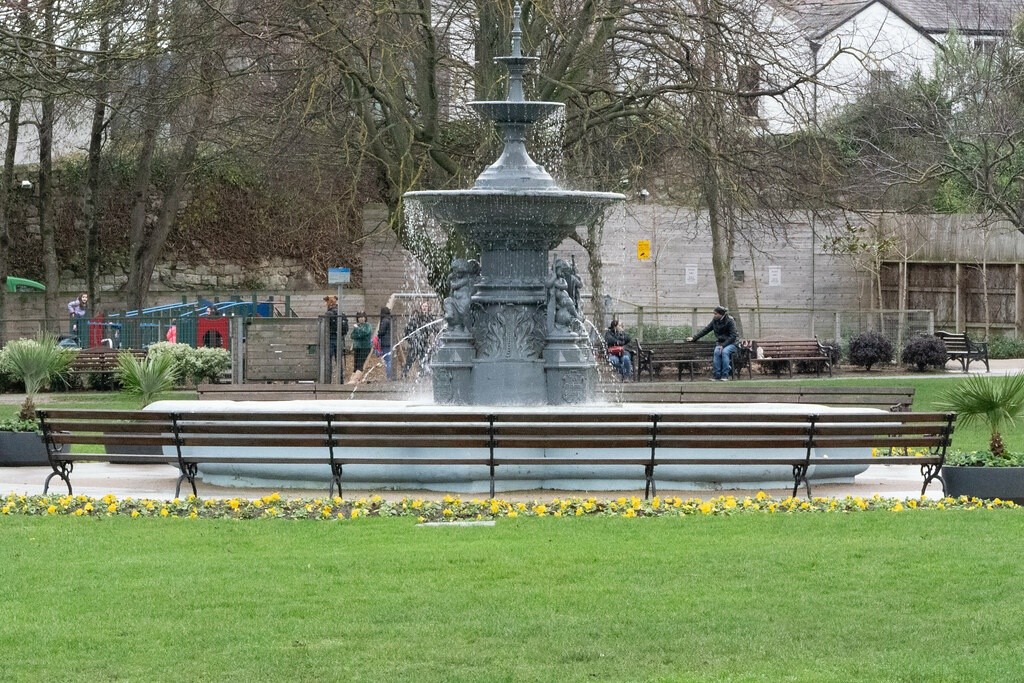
xmin=102 ymin=347 xmax=186 ymax=465
xmin=941 ymin=363 xmax=1024 ymax=502
xmin=0 ymin=333 xmax=75 ymax=467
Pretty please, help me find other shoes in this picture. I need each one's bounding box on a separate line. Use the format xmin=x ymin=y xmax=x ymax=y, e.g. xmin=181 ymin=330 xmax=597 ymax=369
xmin=720 ymin=375 xmax=728 ymax=381
xmin=709 ymin=374 xmax=721 ymax=381
xmin=617 ymin=374 xmax=623 ymax=382
xmin=623 ymin=372 xmax=634 ymax=383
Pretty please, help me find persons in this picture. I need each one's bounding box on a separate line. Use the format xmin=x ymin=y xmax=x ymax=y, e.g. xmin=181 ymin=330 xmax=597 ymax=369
xmin=205 ymin=306 xmax=221 ymax=347
xmin=68 ymin=293 xmax=88 ymax=330
xmin=375 ymin=307 xmax=393 ymax=380
xmin=443 ymin=259 xmax=480 ymax=331
xmin=686 ymin=306 xmax=739 ymax=381
xmin=166 ymin=319 xmax=176 ymax=343
xmin=605 ymin=320 xmax=633 ymax=382
xmin=326 ymin=297 xmax=349 ymax=384
xmin=544 ymin=259 xmax=589 ymax=322
xmin=350 ymin=311 xmax=372 ymax=371
xmin=401 ymin=302 xmax=439 ymax=378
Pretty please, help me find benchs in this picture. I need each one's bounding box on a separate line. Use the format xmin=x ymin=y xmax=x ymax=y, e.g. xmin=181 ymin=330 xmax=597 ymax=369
xmin=37 ymin=409 xmax=958 ymax=503
xmin=64 ymin=349 xmax=147 ymax=392
xmin=594 ymin=339 xmax=636 ymax=381
xmin=635 ymin=335 xmax=737 ymax=378
xmin=739 ymin=335 xmax=833 ymax=377
xmin=196 ymin=383 xmax=916 ymax=458
xmin=934 ymin=331 xmax=990 ymax=373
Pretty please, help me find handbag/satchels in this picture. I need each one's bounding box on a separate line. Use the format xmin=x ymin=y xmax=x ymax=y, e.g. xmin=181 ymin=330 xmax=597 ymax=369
xmin=608 ymin=346 xmax=624 ymax=356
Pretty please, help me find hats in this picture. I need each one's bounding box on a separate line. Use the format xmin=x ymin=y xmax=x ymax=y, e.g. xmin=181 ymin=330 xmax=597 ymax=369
xmin=714 ymin=306 xmax=728 ymax=316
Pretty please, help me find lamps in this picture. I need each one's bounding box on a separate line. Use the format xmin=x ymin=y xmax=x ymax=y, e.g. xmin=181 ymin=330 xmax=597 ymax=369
xmin=19 ymin=179 xmax=33 ymax=189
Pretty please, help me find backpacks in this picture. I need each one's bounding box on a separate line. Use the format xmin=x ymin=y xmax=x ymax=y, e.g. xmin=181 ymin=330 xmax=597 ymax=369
xmin=330 ymin=312 xmax=348 ymax=337
xmin=371 ymin=336 xmax=383 ymax=359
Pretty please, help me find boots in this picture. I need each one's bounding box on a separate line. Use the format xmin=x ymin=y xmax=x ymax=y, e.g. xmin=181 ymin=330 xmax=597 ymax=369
xmin=344 ymin=370 xmax=363 ymax=385
